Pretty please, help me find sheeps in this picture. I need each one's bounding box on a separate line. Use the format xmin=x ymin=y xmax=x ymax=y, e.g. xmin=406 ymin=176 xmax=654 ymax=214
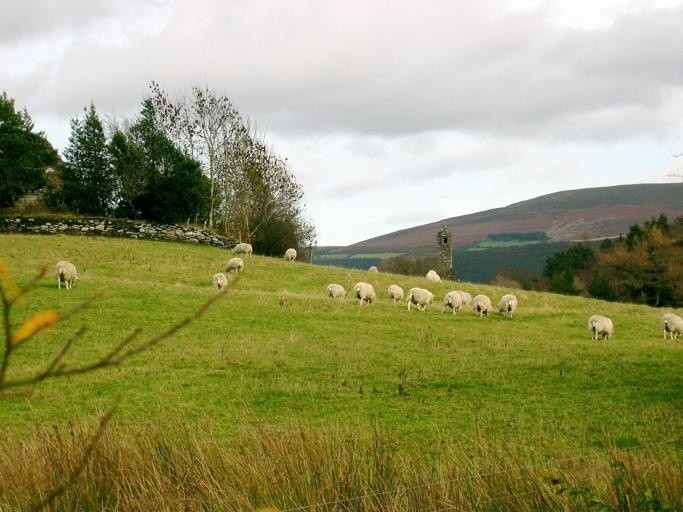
xmin=212 ymin=273 xmax=228 ymax=291
xmin=54 ymin=260 xmax=81 ymax=290
xmin=368 ymin=266 xmax=378 ymax=272
xmin=231 ymin=243 xmax=253 ymax=259
xmin=425 ymin=269 xmax=441 ymax=282
xmin=225 ymin=258 xmax=244 ymax=273
xmin=327 ymin=282 xmax=518 ymax=318
xmin=660 ymin=313 xmax=683 ymax=340
xmin=283 ymin=248 xmax=297 ymax=263
xmin=588 ymin=315 xmax=613 ymax=341
